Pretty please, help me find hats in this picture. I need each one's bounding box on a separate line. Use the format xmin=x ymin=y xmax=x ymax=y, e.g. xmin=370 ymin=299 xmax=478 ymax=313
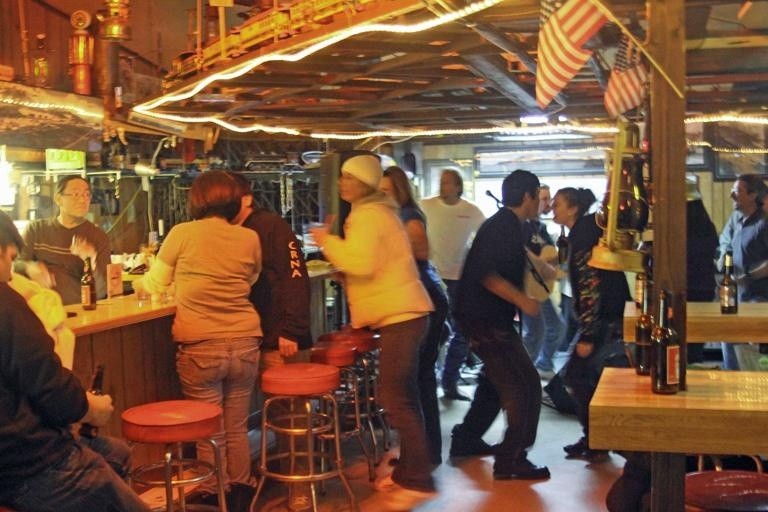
xmin=340 ymin=154 xmax=381 ymax=190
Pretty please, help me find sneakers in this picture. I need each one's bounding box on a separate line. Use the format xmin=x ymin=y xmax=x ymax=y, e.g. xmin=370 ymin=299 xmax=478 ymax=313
xmin=202 ymin=484 xmax=255 ymax=512
xmin=444 ymin=386 xmax=472 ymax=403
xmin=536 ymin=368 xmax=556 ymax=381
xmin=564 ymin=435 xmax=609 ymax=456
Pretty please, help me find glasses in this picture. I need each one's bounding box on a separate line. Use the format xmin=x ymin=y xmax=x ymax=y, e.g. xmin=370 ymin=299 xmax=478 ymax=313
xmin=60 ymin=191 xmax=93 ymax=199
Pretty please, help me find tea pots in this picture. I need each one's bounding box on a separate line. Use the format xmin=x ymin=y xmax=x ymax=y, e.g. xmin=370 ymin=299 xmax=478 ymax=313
xmin=94 ymin=0 xmax=134 ymax=43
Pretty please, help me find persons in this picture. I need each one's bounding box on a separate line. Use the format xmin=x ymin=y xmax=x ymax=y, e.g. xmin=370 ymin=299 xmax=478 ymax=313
xmin=644 ymin=167 xmax=767 ymax=304
xmin=309 ymin=153 xmax=634 ymax=512
xmin=0 ymin=169 xmax=309 ymax=511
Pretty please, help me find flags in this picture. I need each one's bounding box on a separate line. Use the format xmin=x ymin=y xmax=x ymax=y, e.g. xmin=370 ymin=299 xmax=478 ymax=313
xmin=600 ymin=35 xmax=652 ymax=115
xmin=535 ymin=0 xmax=611 ymax=109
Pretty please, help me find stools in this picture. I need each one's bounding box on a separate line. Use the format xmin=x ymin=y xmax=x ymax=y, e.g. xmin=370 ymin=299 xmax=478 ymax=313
xmin=250 ymin=362 xmax=357 ymax=512
xmin=310 ymin=320 xmax=389 ymax=482
xmin=122 ymin=401 xmax=228 ymax=512
xmin=684 ymin=470 xmax=768 ymax=512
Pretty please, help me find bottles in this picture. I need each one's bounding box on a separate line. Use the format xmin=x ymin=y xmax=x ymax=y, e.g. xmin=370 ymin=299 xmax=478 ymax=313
xmin=649 ymin=289 xmax=680 ymax=393
xmin=718 ymin=250 xmax=739 ymax=315
xmin=204 ymin=4 xmax=219 ymax=44
xmin=79 ymin=257 xmax=97 ymax=310
xmin=634 ymin=271 xmax=650 ymax=309
xmin=635 ymin=276 xmax=655 ymax=375
xmin=76 ymin=364 xmax=106 ymax=439
xmin=86 ymin=137 xmax=144 ymax=175
xmin=30 ymin=31 xmax=52 ymax=92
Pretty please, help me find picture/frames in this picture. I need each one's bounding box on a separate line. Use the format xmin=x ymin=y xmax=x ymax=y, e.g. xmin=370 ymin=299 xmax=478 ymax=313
xmin=712 ymin=123 xmax=768 ymax=181
xmin=684 ymin=121 xmax=712 ymax=172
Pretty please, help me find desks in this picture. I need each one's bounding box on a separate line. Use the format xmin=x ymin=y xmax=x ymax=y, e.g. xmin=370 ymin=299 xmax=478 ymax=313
xmin=588 ymin=364 xmax=768 ymax=512
xmin=623 ymin=299 xmax=768 ymax=345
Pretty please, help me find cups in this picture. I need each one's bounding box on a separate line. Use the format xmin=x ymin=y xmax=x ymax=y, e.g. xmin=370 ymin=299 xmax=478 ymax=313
xmin=101 ymin=190 xmax=119 ymax=215
xmin=70 ymin=30 xmax=91 ymax=65
xmin=312 ymin=221 xmax=326 ymax=230
xmin=135 ymin=282 xmax=176 ymax=303
xmin=72 ymin=234 xmax=86 ymax=247
xmin=73 ymin=61 xmax=92 ymax=96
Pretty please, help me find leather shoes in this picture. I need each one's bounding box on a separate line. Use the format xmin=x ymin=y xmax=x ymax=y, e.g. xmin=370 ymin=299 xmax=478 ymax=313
xmin=493 ymin=457 xmax=553 ymax=482
xmin=449 ymin=435 xmax=492 ymax=457
